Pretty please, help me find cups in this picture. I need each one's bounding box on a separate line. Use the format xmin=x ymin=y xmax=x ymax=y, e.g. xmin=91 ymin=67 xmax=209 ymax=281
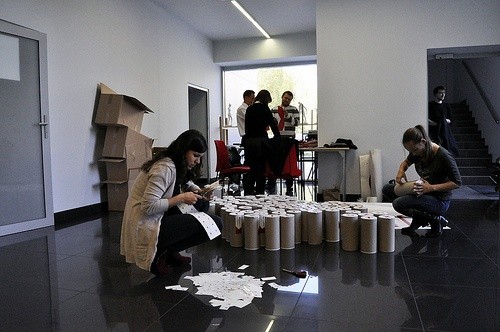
xmin=215 ymin=195 xmax=395 ymax=255
xmin=394 ymin=179 xmax=423 ymax=196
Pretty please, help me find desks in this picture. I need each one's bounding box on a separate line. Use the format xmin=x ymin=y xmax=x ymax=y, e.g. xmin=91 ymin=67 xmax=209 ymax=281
xmin=297 ymin=146 xmax=350 ymax=202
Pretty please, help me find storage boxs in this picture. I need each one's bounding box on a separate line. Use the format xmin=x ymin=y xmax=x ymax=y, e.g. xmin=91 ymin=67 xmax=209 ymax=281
xmin=94 ymin=81 xmax=157 ymax=212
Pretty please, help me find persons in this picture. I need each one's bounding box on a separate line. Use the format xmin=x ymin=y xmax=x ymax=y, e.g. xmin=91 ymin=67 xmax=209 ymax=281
xmin=244 ymin=89 xmax=280 ymax=195
xmin=236 ymin=90 xmax=256 ymax=147
xmin=391 ymin=125 xmax=463 ymax=236
xmin=120 ymin=129 xmax=223 ymax=276
xmin=428 ymin=85 xmax=453 ymax=147
xmin=266 ymin=91 xmax=300 ymax=196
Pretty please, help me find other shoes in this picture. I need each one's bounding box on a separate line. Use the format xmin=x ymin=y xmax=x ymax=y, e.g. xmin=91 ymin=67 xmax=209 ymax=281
xmin=168 ymin=249 xmax=191 ymax=265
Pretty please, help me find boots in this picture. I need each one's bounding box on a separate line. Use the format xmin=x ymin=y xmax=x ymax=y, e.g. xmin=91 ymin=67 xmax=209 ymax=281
xmin=411 ymin=211 xmax=448 ymax=237
xmin=400 ymin=210 xmax=429 ymax=234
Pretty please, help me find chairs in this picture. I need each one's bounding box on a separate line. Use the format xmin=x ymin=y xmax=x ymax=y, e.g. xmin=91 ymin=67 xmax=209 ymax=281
xmin=263 ymin=141 xmax=302 ymax=195
xmin=215 ymin=140 xmax=252 ymax=195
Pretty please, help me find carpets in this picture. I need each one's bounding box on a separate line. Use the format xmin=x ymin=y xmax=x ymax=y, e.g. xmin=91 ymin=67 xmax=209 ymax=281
xmin=467 ymin=184 xmax=500 ymax=197
